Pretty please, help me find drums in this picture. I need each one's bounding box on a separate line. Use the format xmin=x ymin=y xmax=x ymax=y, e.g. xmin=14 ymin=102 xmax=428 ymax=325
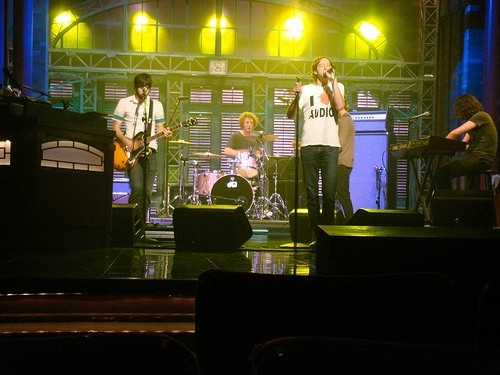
xmin=197 ymin=172 xmax=224 ymax=197
xmin=210 ymin=174 xmax=254 ymax=214
xmin=234 ymin=155 xmax=258 ymax=178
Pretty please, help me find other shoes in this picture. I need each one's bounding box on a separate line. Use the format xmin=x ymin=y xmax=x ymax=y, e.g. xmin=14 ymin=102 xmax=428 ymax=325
xmin=310 ymin=241 xmax=316 ymax=247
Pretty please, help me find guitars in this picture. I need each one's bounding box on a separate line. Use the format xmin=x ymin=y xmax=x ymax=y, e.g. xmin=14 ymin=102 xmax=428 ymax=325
xmin=113 ymin=117 xmax=198 ymax=172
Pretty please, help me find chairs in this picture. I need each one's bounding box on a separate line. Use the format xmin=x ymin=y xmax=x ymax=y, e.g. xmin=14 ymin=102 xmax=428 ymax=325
xmin=0 ymin=271 xmax=500 ymax=375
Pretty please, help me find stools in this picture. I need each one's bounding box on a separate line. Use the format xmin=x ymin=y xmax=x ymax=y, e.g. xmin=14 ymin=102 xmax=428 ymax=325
xmin=468 ymin=169 xmax=492 ymax=192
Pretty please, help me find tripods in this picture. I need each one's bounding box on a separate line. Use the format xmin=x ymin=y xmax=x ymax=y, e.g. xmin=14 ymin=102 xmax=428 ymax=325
xmin=155 ymin=135 xmax=285 ymax=221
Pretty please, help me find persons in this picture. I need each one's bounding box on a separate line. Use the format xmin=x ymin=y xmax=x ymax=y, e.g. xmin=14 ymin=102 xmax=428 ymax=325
xmin=112 ymin=73 xmax=173 ymax=221
xmin=434 ymin=93 xmax=498 ymax=189
xmin=338 ymin=96 xmax=356 ymax=220
xmin=225 ymin=112 xmax=264 ymax=158
xmin=287 ymin=57 xmax=345 ymax=246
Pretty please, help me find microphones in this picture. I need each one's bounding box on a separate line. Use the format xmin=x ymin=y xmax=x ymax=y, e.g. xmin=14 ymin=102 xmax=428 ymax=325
xmin=326 ymin=68 xmax=333 ymax=73
xmin=411 ymin=112 xmax=429 ymax=119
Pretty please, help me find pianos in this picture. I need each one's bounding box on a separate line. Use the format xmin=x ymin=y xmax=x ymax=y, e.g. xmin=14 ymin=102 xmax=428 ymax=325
xmin=390 ymin=134 xmax=467 ymax=226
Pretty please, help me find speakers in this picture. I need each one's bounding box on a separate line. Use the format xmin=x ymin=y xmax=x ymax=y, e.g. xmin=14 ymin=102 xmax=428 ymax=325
xmin=173 ymin=205 xmax=254 ymax=250
xmin=269 ymin=180 xmax=308 ymax=211
xmin=266 ymin=157 xmax=303 ymax=180
xmin=288 ymin=208 xmax=345 ymax=244
xmin=113 ymin=202 xmax=145 ymax=246
xmin=347 ymin=208 xmax=424 ymax=227
xmin=424 ymin=189 xmax=496 ymax=227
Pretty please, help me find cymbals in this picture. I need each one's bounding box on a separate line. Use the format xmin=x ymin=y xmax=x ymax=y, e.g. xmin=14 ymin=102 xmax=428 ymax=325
xmin=188 ymin=152 xmax=226 ymax=158
xmin=167 ymin=141 xmax=198 ymax=146
xmin=244 ymin=134 xmax=278 ymax=142
